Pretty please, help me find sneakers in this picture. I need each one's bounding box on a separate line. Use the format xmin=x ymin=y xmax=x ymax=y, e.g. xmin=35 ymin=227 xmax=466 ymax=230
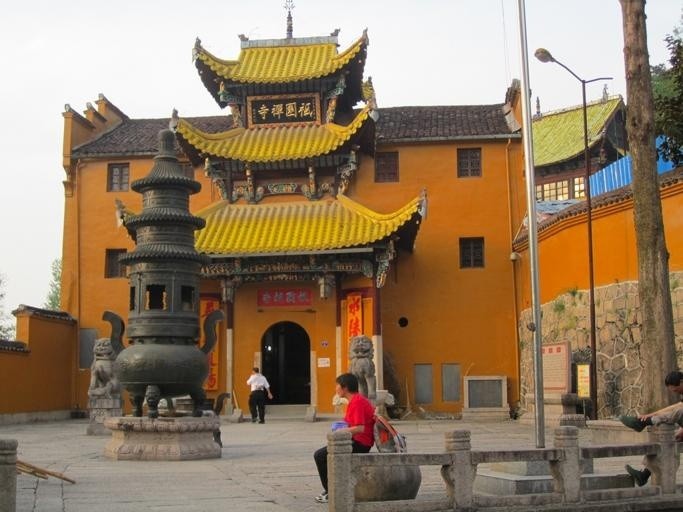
xmin=315 ymin=493 xmax=328 ymax=504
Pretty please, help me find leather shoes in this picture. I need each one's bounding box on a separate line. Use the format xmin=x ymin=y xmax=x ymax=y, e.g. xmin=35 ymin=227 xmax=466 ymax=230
xmin=252 ymin=418 xmax=256 ymax=422
xmin=619 ymin=415 xmax=645 ymax=433
xmin=624 ymin=464 xmax=648 ymax=487
xmin=259 ymin=421 xmax=263 ymax=423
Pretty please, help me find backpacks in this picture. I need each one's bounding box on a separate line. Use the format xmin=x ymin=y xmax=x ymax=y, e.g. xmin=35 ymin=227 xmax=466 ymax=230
xmin=373 ymin=416 xmax=406 ymax=454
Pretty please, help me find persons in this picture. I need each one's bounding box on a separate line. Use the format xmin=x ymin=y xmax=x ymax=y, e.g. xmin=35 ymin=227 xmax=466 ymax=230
xmin=244 ymin=367 xmax=272 ymax=424
xmin=313 ymin=372 xmax=376 ymax=503
xmin=618 ymin=370 xmax=683 ymax=487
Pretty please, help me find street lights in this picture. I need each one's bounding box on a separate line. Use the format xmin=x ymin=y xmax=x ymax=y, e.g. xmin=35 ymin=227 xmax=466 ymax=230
xmin=533 ymin=47 xmax=601 ymax=422
